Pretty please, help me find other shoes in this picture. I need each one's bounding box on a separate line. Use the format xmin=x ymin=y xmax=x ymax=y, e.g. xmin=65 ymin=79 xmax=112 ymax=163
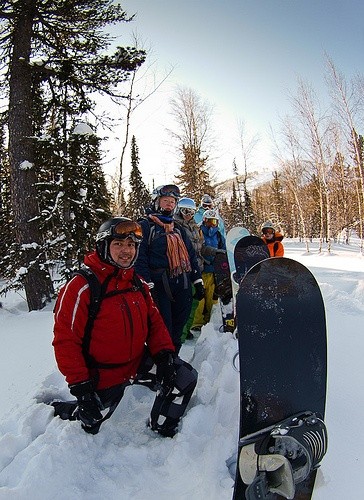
xmin=186 ymin=332 xmax=193 ymax=339
xmin=194 ymin=326 xmax=200 ymax=331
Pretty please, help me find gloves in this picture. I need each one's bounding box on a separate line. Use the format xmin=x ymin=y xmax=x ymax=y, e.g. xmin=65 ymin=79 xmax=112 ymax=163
xmin=153 ymin=350 xmax=176 ymax=388
xmin=69 ymin=380 xmax=104 ymax=424
xmin=193 ymin=282 xmax=205 ymax=301
xmin=150 ymin=288 xmax=158 ymax=301
xmin=212 ymin=248 xmax=226 ymax=255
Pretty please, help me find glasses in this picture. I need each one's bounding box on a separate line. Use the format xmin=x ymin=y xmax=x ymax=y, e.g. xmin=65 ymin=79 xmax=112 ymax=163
xmin=206 ymin=217 xmax=218 ymax=226
xmin=161 ymin=185 xmax=180 ymax=196
xmin=202 ymin=203 xmax=212 ymax=209
xmin=110 ymin=220 xmax=143 ymax=239
xmin=181 ymin=208 xmax=195 ymax=216
xmin=263 ymin=228 xmax=273 ymax=234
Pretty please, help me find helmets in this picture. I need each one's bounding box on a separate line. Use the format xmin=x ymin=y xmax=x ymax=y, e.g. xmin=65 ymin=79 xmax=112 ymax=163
xmin=261 ymin=221 xmax=275 ymax=231
xmin=151 ymin=185 xmax=178 ymax=205
xmin=177 ymin=198 xmax=196 ymax=211
xmin=201 ymin=194 xmax=213 ymax=208
xmin=202 ymin=210 xmax=217 ymax=221
xmin=95 ymin=217 xmax=142 ymax=246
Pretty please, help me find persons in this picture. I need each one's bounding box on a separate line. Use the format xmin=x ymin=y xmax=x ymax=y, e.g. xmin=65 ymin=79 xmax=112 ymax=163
xmin=132 ymin=184 xmax=204 ymax=354
xmin=260 ymin=219 xmax=285 ymax=258
xmin=47 ymin=218 xmax=198 ymax=438
xmin=193 ymin=193 xmax=224 ymax=236
xmin=192 ymin=210 xmax=226 ymax=332
xmin=173 ymin=198 xmax=205 ymax=344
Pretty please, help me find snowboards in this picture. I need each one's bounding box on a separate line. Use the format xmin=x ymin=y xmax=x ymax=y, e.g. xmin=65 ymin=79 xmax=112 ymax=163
xmin=232 ymin=257 xmax=328 ymax=499
xmin=225 ymin=226 xmax=251 ymax=321
xmin=234 ymin=236 xmax=270 ymax=285
xmin=213 ymin=252 xmax=237 ymax=333
